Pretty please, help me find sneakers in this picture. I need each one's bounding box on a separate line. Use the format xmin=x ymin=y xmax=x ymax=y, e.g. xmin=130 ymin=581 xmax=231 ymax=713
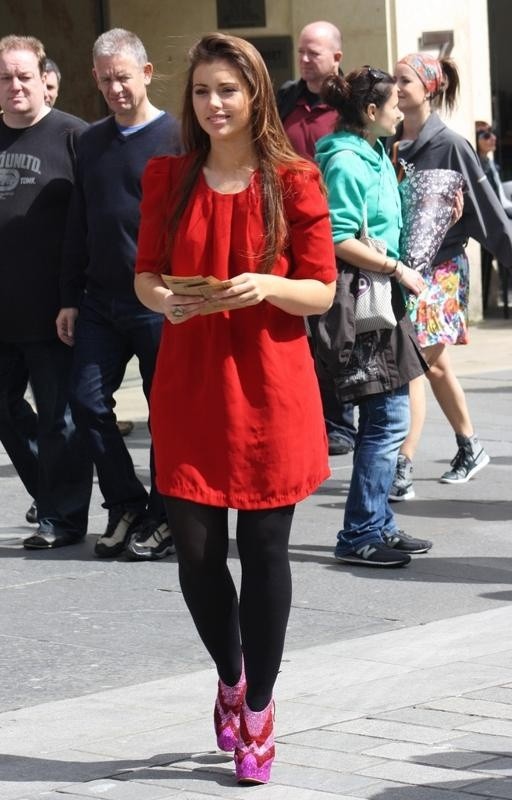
xmin=213 ymin=651 xmax=247 ymax=751
xmin=117 ymin=421 xmax=133 ymax=434
xmin=95 ymin=508 xmax=147 ymax=556
xmin=384 ymin=531 xmax=432 ymax=555
xmin=328 ymin=436 xmax=352 ymax=455
xmin=441 ymin=434 xmax=489 ymax=483
xmin=387 ymin=454 xmax=415 ymax=503
xmin=24 ymin=520 xmax=84 ymax=550
xmin=234 ymin=696 xmax=275 ymax=784
xmin=336 ymin=542 xmax=410 ymax=569
xmin=26 ymin=500 xmax=38 ymax=522
xmin=125 ymin=522 xmax=176 ymax=559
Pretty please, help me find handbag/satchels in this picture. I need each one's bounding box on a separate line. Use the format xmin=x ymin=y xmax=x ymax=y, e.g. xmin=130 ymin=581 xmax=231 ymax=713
xmin=354 ymin=238 xmax=397 ymax=335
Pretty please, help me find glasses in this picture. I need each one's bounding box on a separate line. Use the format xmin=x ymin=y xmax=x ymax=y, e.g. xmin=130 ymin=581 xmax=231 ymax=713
xmin=363 ymin=64 xmax=385 ymax=106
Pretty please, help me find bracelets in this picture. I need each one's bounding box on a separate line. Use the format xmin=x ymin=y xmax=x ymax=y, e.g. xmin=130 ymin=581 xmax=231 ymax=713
xmin=384 ymin=259 xmax=398 ymax=277
xmin=397 ymin=261 xmax=405 ymax=284
xmin=380 ymin=256 xmax=389 ymax=274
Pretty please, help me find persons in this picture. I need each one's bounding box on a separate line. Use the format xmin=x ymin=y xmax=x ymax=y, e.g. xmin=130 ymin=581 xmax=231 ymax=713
xmin=0 ymin=34 xmax=94 ymax=550
xmin=379 ymin=51 xmax=512 ymax=501
xmin=56 ymin=28 xmax=189 ymax=560
xmin=134 ymin=33 xmax=339 ymax=783
xmin=313 ymin=65 xmax=434 ymax=568
xmin=41 ymin=58 xmax=134 ymax=436
xmin=278 ymin=21 xmax=357 ymax=455
xmin=476 ymin=121 xmax=512 ymax=312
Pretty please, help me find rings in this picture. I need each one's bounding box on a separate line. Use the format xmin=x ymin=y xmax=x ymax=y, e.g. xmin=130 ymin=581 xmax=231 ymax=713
xmin=170 ymin=307 xmax=185 ymax=318
xmin=418 ymin=286 xmax=421 ymax=288
